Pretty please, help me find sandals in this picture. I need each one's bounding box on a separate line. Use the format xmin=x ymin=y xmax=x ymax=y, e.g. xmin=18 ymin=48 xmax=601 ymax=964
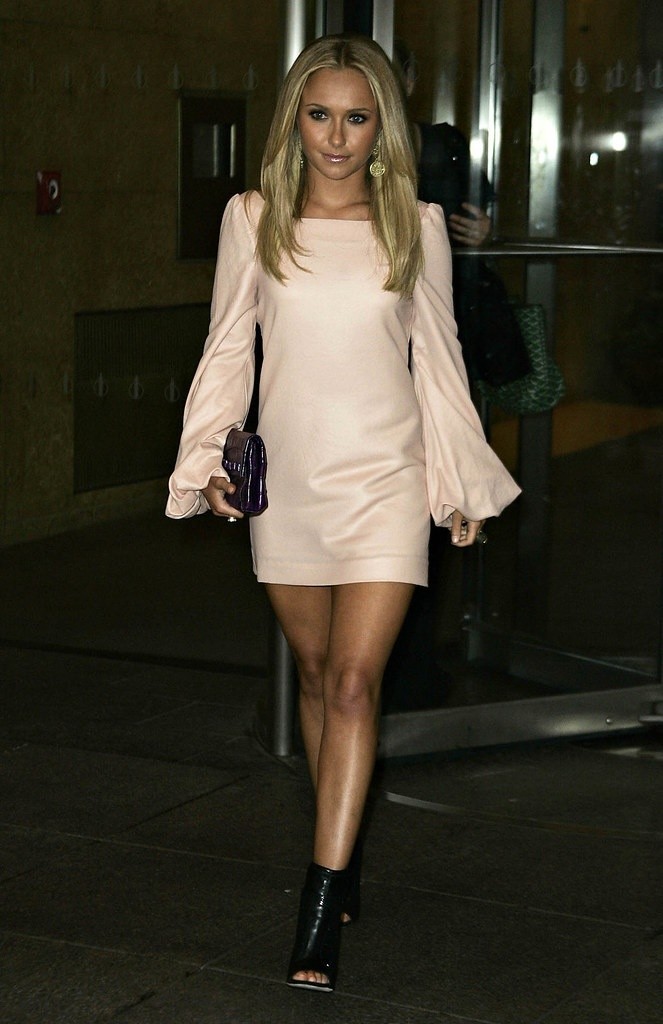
xmin=285 ymin=861 xmax=347 ymax=993
xmin=338 ymin=837 xmax=363 ymax=926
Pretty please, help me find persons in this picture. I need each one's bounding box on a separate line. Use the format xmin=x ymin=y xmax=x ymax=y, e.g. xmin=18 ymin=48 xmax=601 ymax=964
xmin=162 ymin=32 xmax=521 ymax=989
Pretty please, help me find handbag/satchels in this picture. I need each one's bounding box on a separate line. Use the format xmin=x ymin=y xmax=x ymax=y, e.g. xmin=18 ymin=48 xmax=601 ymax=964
xmin=470 ymin=300 xmax=567 ymax=417
xmin=466 ymin=283 xmax=532 ymax=388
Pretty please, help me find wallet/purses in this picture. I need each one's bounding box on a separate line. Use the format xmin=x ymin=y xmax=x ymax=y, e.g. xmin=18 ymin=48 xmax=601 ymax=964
xmin=222 ymin=428 xmax=268 ymax=516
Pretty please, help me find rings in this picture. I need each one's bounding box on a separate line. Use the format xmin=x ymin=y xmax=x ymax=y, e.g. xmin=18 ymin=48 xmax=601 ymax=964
xmin=227 ymin=517 xmax=236 ymax=522
xmin=476 ymin=531 xmax=486 ymax=543
